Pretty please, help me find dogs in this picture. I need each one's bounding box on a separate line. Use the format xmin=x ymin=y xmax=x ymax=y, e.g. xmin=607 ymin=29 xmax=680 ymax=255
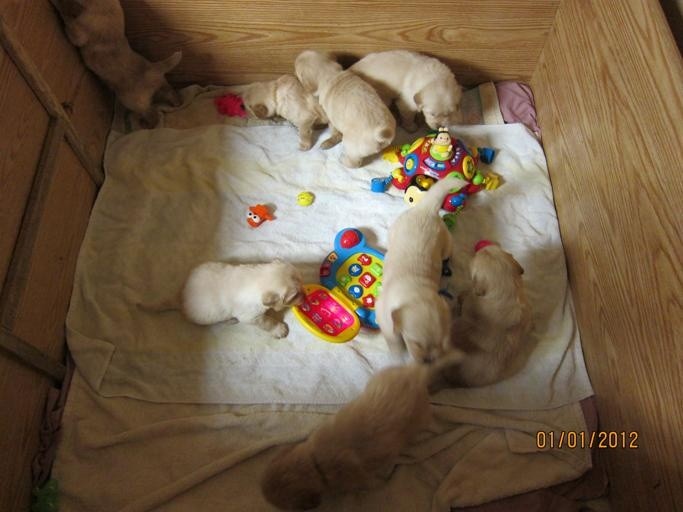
xmin=50 ymin=0 xmax=184 ymax=128
xmin=261 ymin=350 xmax=469 ymax=512
xmin=223 ymin=51 xmax=463 ymax=168
xmin=375 ymin=177 xmax=532 ymax=387
xmin=124 ymin=259 xmax=312 ymax=339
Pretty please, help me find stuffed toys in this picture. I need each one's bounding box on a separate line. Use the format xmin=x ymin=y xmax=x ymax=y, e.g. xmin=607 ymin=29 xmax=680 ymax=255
xmin=246 ymin=203 xmax=272 ymax=229
